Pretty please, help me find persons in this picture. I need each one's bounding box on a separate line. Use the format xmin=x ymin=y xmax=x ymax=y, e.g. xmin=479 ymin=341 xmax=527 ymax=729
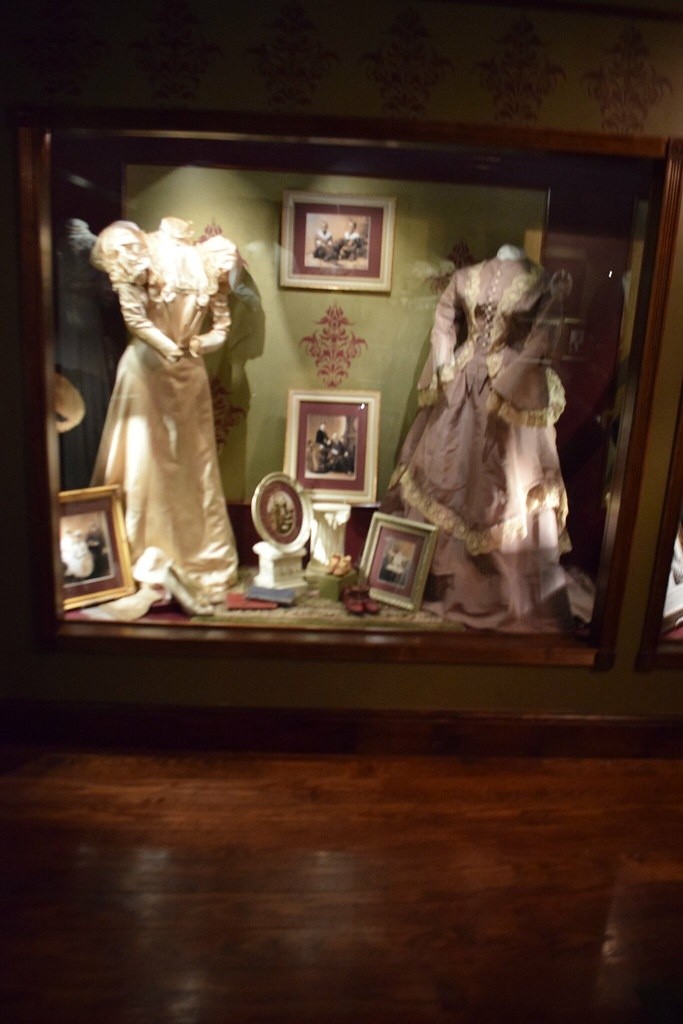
xmin=338 ymin=221 xmax=364 ymax=261
xmin=90 ymin=213 xmax=239 ymax=603
xmin=63 ymin=521 xmax=105 ymax=580
xmin=306 ymin=422 xmax=356 ymax=474
xmin=380 ymin=542 xmax=407 ymax=583
xmin=315 ymin=221 xmax=338 ymax=262
xmin=390 ymin=246 xmax=571 ymax=613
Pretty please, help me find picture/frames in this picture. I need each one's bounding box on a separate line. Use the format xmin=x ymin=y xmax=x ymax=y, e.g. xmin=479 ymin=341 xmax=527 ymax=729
xmin=58 ymin=485 xmax=137 ymax=613
xmin=280 ymin=189 xmax=398 ymax=293
xmin=359 ymin=511 xmax=441 ymax=611
xmin=283 ymin=390 xmax=380 ymax=505
